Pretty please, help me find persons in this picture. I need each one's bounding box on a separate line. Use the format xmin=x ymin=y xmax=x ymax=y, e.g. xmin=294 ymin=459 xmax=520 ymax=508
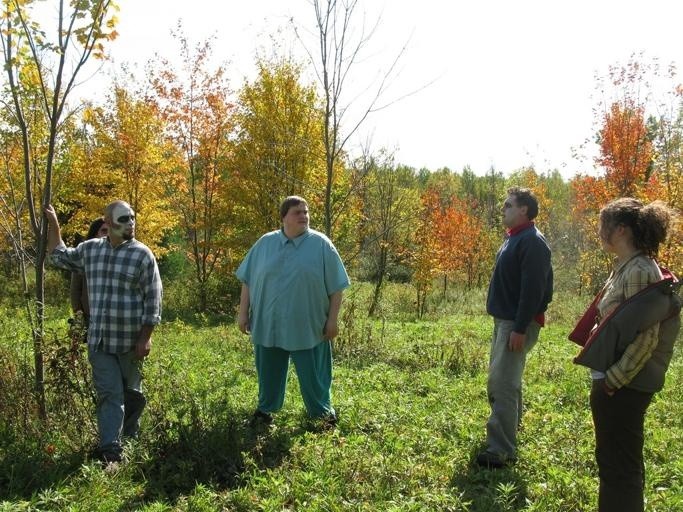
xmin=564 ymin=195 xmax=683 ymax=511
xmin=40 ymin=199 xmax=164 ymax=463
xmin=232 ymin=194 xmax=353 ymax=433
xmin=69 ymin=216 xmax=107 ymax=344
xmin=474 ymin=183 xmax=555 ymax=468
